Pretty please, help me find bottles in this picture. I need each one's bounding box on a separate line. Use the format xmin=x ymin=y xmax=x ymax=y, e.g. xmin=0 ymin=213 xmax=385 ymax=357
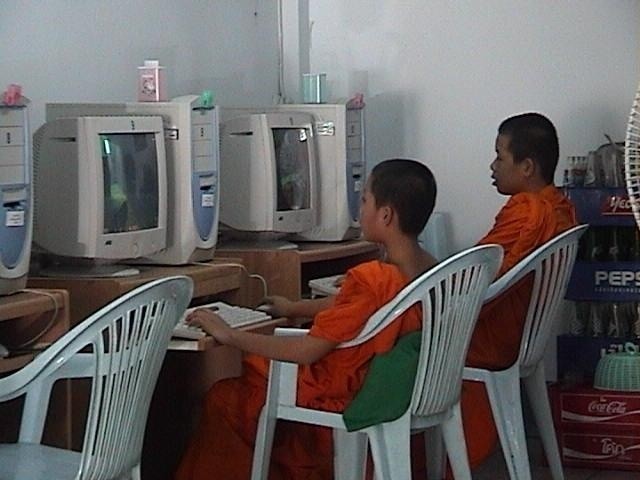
xmin=588 ymin=227 xmax=640 ymax=260
xmin=563 ymin=151 xmax=604 ymax=189
xmin=568 ymin=300 xmax=640 ymax=339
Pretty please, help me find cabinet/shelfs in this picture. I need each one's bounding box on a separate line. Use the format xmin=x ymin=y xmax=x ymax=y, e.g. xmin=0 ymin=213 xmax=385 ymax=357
xmin=548 ymin=186 xmax=638 ymax=473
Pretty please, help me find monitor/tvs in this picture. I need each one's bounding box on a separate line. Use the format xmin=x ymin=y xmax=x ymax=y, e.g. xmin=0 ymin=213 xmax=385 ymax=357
xmin=35 ymin=117 xmax=171 ymax=277
xmin=221 ymin=112 xmax=315 ymax=250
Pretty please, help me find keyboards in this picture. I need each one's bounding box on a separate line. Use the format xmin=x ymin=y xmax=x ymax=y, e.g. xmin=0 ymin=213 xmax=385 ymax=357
xmin=171 ymin=303 xmax=271 ymax=343
xmin=310 ymin=275 xmax=348 ymax=296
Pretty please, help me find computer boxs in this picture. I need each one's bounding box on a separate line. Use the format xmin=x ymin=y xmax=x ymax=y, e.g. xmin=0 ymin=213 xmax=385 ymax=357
xmin=1 ymin=102 xmax=34 ymax=296
xmin=128 ymin=96 xmax=222 ymax=265
xmin=258 ymin=104 xmax=364 ymax=245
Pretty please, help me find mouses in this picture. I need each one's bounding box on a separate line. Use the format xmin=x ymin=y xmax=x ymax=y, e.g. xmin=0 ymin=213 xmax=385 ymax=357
xmin=254 ymin=302 xmax=273 ymax=313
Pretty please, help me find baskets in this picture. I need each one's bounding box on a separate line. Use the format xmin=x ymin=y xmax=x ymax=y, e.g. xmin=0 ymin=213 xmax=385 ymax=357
xmin=594 ymin=352 xmax=640 ymax=391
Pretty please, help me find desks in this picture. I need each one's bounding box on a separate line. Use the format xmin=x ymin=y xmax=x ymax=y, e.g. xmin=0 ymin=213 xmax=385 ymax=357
xmin=2 ymin=285 xmax=74 ymax=479
xmin=212 ymin=233 xmax=391 ymax=332
xmin=26 ymin=254 xmax=291 ymax=477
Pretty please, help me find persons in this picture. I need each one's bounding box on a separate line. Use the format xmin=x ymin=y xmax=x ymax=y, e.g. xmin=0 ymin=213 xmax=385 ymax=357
xmin=175 ymin=157 xmax=440 ymax=480
xmin=391 ymin=110 xmax=572 ymax=479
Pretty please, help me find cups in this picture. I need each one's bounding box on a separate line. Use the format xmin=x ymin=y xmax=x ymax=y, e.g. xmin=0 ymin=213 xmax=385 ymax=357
xmin=302 ymin=73 xmax=327 ymax=103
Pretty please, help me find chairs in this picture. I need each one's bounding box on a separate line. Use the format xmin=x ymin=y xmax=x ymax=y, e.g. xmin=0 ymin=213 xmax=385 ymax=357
xmin=249 ymin=241 xmax=505 ymax=478
xmin=421 ymin=222 xmax=592 ymax=480
xmin=3 ymin=273 xmax=195 ymax=479
